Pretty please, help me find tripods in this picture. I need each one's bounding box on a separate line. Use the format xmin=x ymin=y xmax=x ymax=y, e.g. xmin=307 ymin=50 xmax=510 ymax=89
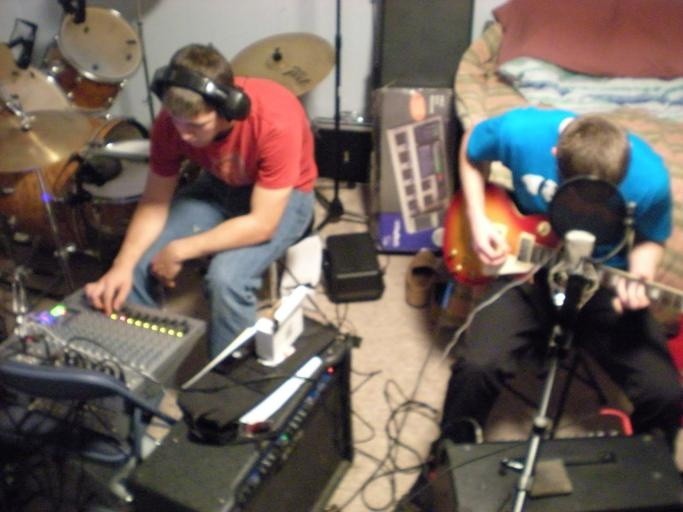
xmin=314 ymin=0 xmax=376 ymax=233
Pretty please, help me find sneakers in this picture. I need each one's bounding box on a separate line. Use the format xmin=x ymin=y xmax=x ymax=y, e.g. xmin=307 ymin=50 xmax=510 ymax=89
xmin=406 ymin=248 xmax=452 ymax=326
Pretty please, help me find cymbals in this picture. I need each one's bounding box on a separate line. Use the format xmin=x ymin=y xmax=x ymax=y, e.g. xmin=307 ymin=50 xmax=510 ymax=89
xmin=1 ymin=107 xmax=95 ymax=175
xmin=231 ymin=31 xmax=336 ymax=100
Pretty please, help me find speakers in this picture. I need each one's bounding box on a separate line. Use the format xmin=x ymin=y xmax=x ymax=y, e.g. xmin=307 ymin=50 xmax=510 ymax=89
xmin=375 ymin=0 xmax=476 ymax=89
xmin=392 ymin=429 xmax=683 ymax=512
xmin=123 ymin=314 xmax=356 ymax=512
xmin=326 ymin=234 xmax=384 ymax=305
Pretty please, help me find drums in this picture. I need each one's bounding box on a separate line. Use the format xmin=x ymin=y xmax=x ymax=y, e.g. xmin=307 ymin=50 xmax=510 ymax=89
xmin=76 ymin=156 xmax=150 ymax=242
xmin=46 ymin=6 xmax=144 ymax=115
xmin=15 ymin=115 xmax=152 ymax=240
xmin=1 ymin=40 xmax=69 ymax=114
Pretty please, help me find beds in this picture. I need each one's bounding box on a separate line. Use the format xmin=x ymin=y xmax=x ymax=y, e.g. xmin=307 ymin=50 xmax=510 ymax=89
xmin=450 ymin=1 xmax=682 ymax=334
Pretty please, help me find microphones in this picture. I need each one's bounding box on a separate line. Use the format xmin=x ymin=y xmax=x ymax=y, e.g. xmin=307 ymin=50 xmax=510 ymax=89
xmin=69 ymin=148 xmax=123 ymax=187
xmin=546 ymin=179 xmax=630 ymax=301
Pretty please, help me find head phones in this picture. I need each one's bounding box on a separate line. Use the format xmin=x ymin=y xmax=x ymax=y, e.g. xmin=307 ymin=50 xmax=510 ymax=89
xmin=150 ymin=65 xmax=251 ymax=123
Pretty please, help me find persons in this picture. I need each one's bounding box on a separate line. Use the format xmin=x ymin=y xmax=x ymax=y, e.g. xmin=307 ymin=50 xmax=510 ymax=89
xmin=77 ymin=42 xmax=320 ymax=377
xmin=426 ymin=104 xmax=683 ymax=459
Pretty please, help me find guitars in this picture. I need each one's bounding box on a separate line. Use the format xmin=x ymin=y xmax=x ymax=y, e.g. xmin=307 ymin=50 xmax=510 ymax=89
xmin=443 ymin=179 xmax=682 ymax=315
